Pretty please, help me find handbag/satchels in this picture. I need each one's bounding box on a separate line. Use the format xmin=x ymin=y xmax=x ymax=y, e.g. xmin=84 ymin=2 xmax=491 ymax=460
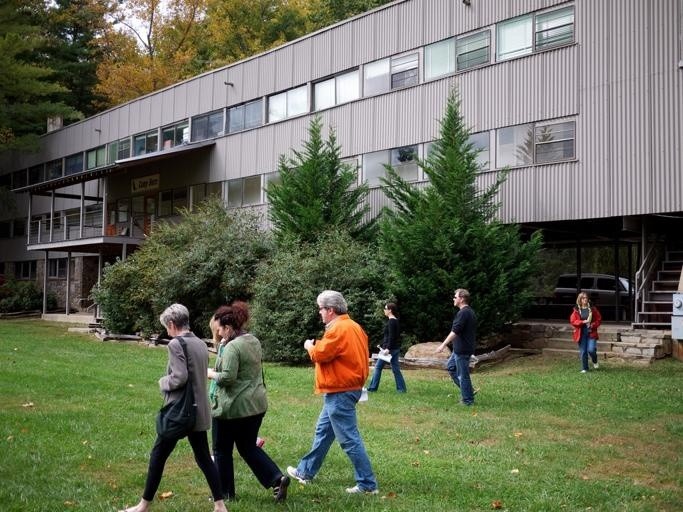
xmin=155 ymin=381 xmax=198 ymax=444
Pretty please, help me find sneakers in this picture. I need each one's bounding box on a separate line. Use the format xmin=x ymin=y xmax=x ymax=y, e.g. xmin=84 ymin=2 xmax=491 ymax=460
xmin=580 ymin=369 xmax=588 ymax=374
xmin=269 ymin=475 xmax=290 ymax=505
xmin=594 ymin=362 xmax=600 ymax=370
xmin=286 ymin=464 xmax=314 ymax=487
xmin=346 ymin=484 xmax=379 ymax=497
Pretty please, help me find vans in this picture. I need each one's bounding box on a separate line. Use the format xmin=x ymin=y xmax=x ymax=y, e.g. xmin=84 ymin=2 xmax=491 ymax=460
xmin=553 ymin=272 xmax=642 ymax=305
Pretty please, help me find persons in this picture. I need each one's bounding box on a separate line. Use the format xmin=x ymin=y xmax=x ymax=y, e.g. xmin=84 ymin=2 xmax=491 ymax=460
xmin=365 ymin=303 xmax=407 ymax=394
xmin=569 ymin=291 xmax=602 ymax=373
xmin=285 ymin=291 xmax=378 ymax=495
xmin=113 ymin=301 xmax=290 ymax=512
xmin=430 ymin=288 xmax=479 ymax=407
